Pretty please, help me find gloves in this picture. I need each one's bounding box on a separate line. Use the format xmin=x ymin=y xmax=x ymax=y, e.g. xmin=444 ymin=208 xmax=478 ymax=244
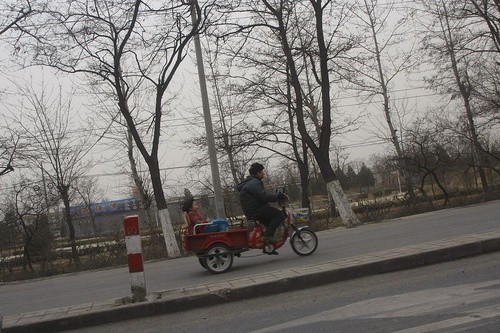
xmin=278 ymin=193 xmax=286 ymax=200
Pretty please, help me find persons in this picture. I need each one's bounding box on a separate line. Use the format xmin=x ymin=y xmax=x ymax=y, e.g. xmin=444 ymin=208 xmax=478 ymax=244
xmin=236 ymin=163 xmax=285 ymax=255
xmin=183 ymin=198 xmax=227 ymax=232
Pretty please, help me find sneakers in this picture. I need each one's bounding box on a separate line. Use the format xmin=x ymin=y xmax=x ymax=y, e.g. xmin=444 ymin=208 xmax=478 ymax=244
xmin=263 ymin=250 xmax=279 ymax=255
xmin=264 ymin=231 xmax=274 ymax=237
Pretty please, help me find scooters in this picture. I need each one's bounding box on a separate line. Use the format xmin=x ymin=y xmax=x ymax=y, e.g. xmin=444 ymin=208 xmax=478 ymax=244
xmin=181 ymin=185 xmax=318 ymax=274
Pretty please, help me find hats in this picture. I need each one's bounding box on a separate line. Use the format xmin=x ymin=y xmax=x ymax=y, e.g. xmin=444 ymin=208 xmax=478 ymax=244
xmin=249 ymin=163 xmax=264 ymax=175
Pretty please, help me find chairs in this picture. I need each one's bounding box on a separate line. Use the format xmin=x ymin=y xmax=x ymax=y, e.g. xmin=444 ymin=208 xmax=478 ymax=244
xmin=182 ymin=211 xmax=207 ymax=234
